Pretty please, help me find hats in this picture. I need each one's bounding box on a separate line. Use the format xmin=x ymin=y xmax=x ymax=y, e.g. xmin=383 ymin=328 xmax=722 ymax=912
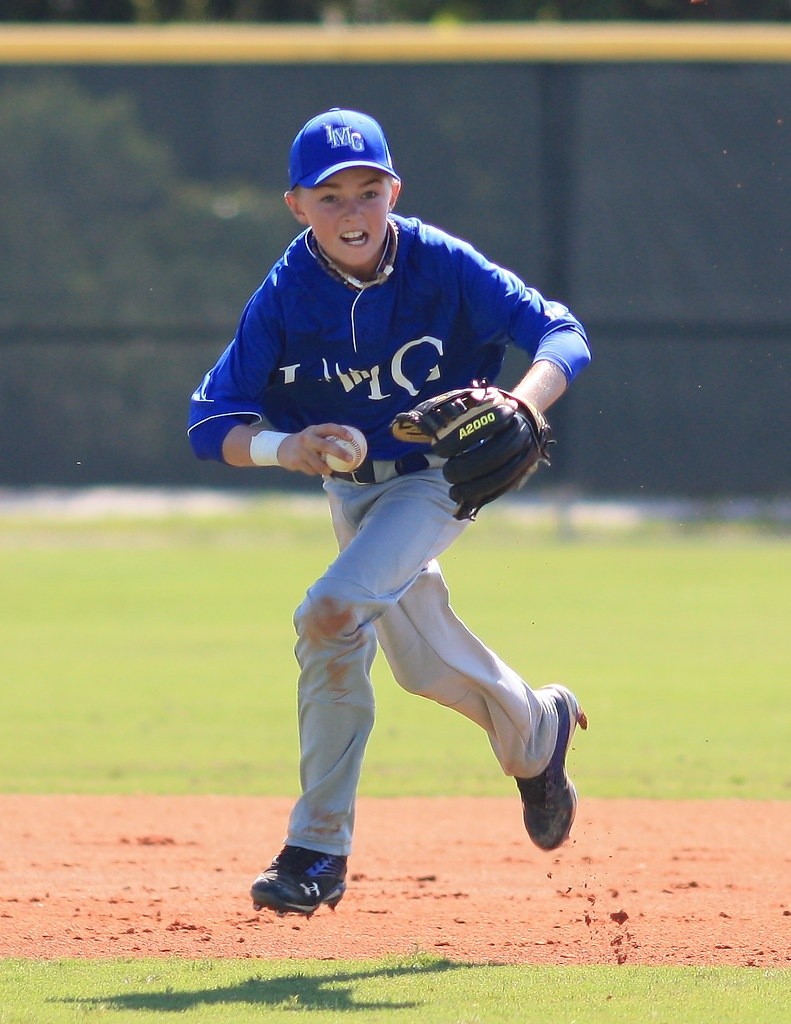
xmin=288 ymin=102 xmax=401 ymax=192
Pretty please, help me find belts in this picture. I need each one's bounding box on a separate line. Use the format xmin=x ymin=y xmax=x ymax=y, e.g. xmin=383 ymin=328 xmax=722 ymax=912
xmin=329 ymin=453 xmax=429 ymax=485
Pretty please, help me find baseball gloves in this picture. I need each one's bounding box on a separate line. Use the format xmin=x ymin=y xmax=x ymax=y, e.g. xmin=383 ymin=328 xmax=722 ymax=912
xmin=388 ymin=378 xmax=556 ymax=521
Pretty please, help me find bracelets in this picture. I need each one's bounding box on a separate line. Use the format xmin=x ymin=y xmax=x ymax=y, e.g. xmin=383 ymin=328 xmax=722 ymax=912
xmin=250 ymin=431 xmax=292 ymax=467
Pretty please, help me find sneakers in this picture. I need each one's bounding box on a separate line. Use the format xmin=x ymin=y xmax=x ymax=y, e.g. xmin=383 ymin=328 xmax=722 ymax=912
xmin=250 ymin=845 xmax=349 ymax=919
xmin=513 ymin=684 xmax=581 ymax=851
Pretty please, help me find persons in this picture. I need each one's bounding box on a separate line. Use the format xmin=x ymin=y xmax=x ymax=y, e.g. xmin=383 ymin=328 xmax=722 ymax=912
xmin=187 ymin=107 xmax=591 ymax=915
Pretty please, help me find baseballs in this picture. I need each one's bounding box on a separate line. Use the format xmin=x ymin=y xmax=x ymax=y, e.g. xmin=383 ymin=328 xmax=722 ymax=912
xmin=321 ymin=425 xmax=368 ymax=472
